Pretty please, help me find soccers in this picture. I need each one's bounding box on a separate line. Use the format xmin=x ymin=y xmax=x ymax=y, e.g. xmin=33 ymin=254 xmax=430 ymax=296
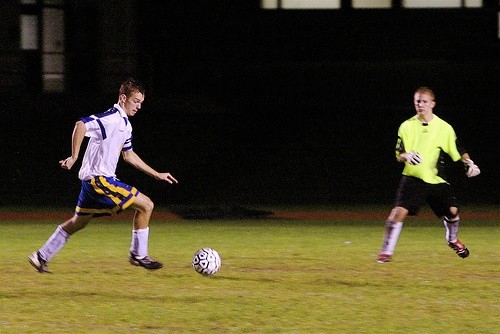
xmin=192 ymin=247 xmax=221 ymax=276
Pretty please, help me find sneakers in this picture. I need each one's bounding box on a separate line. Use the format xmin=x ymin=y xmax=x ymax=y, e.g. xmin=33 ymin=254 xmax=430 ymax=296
xmin=130 ymin=252 xmax=164 ymax=270
xmin=27 ymin=252 xmax=55 ymax=275
xmin=376 ymin=241 xmax=403 ymax=263
xmin=444 ymin=240 xmax=469 ymax=258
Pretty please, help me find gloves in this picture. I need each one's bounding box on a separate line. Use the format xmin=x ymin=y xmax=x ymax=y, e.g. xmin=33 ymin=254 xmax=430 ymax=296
xmin=402 ymin=151 xmax=421 ymax=166
xmin=463 ymin=157 xmax=481 ymax=177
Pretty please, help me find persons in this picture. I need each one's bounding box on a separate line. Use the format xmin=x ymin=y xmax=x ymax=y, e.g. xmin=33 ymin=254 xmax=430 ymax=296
xmin=377 ymin=88 xmax=480 ymax=263
xmin=27 ymin=78 xmax=178 ymax=273
